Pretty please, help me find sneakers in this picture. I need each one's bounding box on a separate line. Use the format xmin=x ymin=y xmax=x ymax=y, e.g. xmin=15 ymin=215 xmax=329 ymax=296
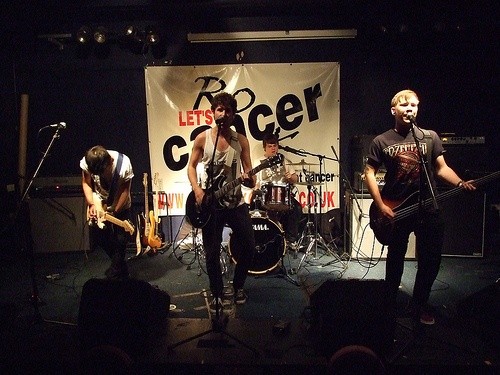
xmin=210 ymin=294 xmax=223 ymax=313
xmin=235 ymin=289 xmax=247 ymax=304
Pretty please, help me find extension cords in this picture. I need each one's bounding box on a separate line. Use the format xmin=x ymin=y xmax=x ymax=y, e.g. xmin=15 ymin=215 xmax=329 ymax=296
xmin=272 ymin=318 xmax=291 ymax=336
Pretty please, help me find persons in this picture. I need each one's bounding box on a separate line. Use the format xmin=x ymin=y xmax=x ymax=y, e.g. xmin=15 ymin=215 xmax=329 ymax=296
xmin=364 ymin=90 xmax=477 ymax=324
xmin=188 ymin=92 xmax=257 ymax=313
xmin=80 ymin=145 xmax=134 ymax=280
xmin=249 ymin=133 xmax=302 ymax=252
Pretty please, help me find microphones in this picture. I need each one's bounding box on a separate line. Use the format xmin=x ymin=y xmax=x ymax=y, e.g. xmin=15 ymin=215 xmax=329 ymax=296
xmin=407 ymin=111 xmax=417 ymax=126
xmin=220 ymin=115 xmax=228 ymax=129
xmin=278 ymin=146 xmax=293 ymax=152
xmin=51 ymin=122 xmax=67 ymax=130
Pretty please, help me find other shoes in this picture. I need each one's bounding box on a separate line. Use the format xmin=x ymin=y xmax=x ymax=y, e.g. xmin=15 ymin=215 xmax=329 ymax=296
xmin=413 ymin=308 xmax=434 ymax=325
xmin=106 ymin=262 xmax=130 ymax=281
xmin=384 ymin=285 xmax=398 ymax=300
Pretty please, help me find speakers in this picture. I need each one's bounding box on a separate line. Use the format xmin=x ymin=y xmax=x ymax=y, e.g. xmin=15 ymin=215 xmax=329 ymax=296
xmin=349 ymin=193 xmax=418 ymax=261
xmin=308 ymin=277 xmax=396 ymax=356
xmin=427 ymin=187 xmax=486 ymax=258
xmin=29 ymin=196 xmax=90 ymax=253
xmin=76 ymin=277 xmax=171 ymax=355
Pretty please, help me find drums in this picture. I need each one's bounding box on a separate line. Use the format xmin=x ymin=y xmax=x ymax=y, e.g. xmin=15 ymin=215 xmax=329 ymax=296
xmin=228 ymin=217 xmax=286 ymax=274
xmin=260 ymin=184 xmax=290 ymax=212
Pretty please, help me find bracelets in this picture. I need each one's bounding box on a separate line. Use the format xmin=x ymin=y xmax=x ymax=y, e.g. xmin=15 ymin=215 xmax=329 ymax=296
xmin=89 ymin=202 xmax=94 ymax=209
xmin=457 ymin=181 xmax=462 ymax=187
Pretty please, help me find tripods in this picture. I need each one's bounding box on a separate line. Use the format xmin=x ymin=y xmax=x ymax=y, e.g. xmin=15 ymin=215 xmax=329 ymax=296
xmin=165 ymin=126 xmax=262 ymax=358
xmin=293 ymin=167 xmax=332 ymax=259
xmin=292 ymin=150 xmax=348 ymax=275
xmin=385 ymin=123 xmax=480 ymax=363
xmin=0 ymin=129 xmax=80 ymax=347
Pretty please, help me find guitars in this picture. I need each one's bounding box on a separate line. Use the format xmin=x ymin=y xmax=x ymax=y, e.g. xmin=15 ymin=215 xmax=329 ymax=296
xmin=186 ymin=153 xmax=285 ymax=230
xmin=139 ymin=172 xmax=162 ymax=248
xmin=368 ymin=171 xmax=500 ymax=246
xmin=86 ymin=192 xmax=135 ymax=235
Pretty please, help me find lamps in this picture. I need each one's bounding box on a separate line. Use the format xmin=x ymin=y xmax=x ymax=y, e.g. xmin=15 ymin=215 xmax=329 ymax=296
xmin=187 ymin=28 xmax=357 ymax=43
xmin=120 ymin=23 xmax=160 ymax=45
xmin=76 ymin=24 xmax=107 ymax=44
